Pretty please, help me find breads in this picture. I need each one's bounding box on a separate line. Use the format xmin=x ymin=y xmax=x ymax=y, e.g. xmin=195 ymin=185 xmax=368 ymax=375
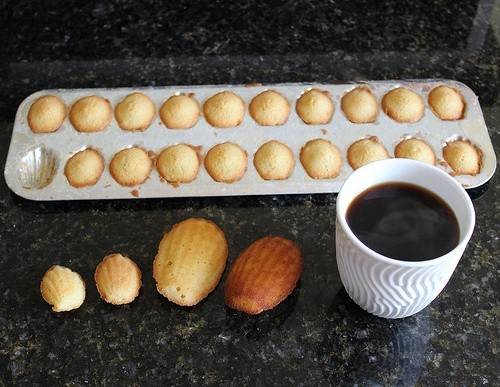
xmin=154 ymin=218 xmax=229 ymax=306
xmin=225 ymin=235 xmax=302 ymax=314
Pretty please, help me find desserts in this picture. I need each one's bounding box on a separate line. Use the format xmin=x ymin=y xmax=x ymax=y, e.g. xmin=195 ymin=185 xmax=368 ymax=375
xmin=29 ymin=85 xmax=482 ymax=187
xmin=40 ymin=264 xmax=86 ymax=312
xmin=95 ymin=253 xmax=142 ymax=305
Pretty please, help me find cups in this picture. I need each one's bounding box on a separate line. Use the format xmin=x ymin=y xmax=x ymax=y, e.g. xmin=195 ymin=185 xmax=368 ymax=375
xmin=335 ymin=157 xmax=476 ymax=318
